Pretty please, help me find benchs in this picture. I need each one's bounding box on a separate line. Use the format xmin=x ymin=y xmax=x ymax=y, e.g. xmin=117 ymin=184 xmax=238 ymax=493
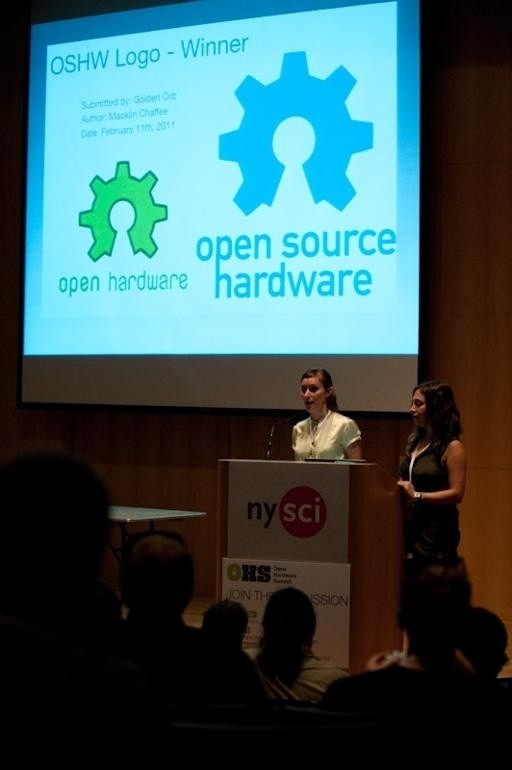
xmin=1 ymin=690 xmax=512 ymax=769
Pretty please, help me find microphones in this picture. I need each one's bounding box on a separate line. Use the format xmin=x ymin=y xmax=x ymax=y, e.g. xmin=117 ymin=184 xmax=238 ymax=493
xmin=265 ymin=412 xmax=311 ymax=460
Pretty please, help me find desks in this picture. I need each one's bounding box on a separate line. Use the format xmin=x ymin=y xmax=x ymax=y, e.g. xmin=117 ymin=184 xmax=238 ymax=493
xmin=100 ymin=503 xmax=207 ymax=606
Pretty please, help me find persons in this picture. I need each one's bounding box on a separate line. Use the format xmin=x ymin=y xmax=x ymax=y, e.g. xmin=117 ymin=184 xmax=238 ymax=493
xmin=290 ymin=368 xmax=363 ymax=462
xmin=0 ymin=449 xmax=511 ymax=767
xmin=395 ymin=379 xmax=467 ymax=555
